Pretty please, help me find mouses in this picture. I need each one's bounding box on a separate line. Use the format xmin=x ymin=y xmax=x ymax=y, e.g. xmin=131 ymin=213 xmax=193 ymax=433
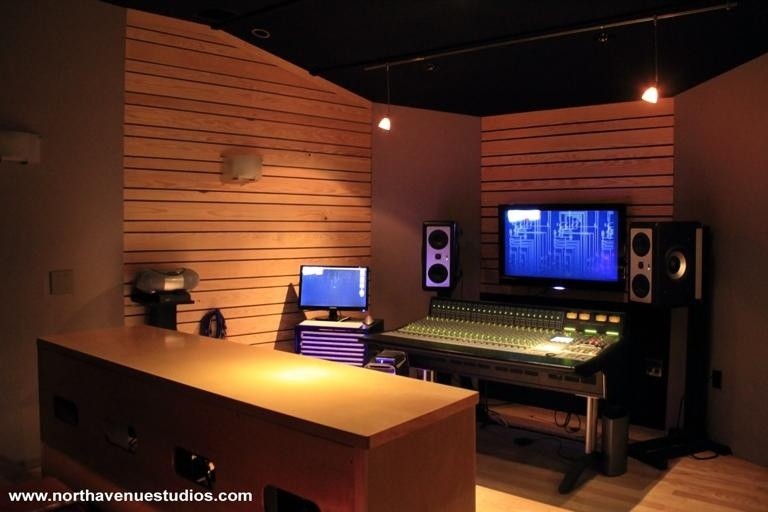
xmin=364 ymin=315 xmax=374 ymax=324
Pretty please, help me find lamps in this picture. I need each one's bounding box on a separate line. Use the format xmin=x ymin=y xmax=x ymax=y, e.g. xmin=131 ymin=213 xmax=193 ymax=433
xmin=377 ymin=63 xmax=391 ymax=130
xmin=0 ymin=130 xmax=40 ymax=162
xmin=222 ymin=150 xmax=260 ymax=183
xmin=641 ymin=18 xmax=659 ymax=104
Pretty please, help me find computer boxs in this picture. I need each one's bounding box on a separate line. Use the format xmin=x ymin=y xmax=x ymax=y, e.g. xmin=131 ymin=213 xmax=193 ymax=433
xmin=364 ymin=353 xmax=408 ymax=377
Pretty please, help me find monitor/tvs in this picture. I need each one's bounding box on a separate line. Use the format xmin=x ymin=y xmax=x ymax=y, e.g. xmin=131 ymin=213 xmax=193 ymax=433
xmin=299 ymin=265 xmax=368 ymax=321
xmin=497 ymin=202 xmax=627 ymax=293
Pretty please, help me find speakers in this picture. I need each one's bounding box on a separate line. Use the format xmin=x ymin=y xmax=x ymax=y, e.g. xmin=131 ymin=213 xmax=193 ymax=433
xmin=628 ymin=220 xmax=704 ymax=305
xmin=421 ymin=219 xmax=457 ymax=292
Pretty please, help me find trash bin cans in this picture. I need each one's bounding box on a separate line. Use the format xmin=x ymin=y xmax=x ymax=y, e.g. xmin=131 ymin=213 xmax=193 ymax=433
xmin=599 ymin=404 xmax=630 ymax=477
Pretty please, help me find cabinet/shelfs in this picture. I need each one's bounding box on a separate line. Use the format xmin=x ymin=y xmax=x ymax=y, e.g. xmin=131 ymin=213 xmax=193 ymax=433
xmin=295 ymin=317 xmax=385 ymax=367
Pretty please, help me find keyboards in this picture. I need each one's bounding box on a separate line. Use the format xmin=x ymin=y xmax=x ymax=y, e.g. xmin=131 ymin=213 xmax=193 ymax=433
xmin=299 ymin=320 xmax=363 ymax=330
